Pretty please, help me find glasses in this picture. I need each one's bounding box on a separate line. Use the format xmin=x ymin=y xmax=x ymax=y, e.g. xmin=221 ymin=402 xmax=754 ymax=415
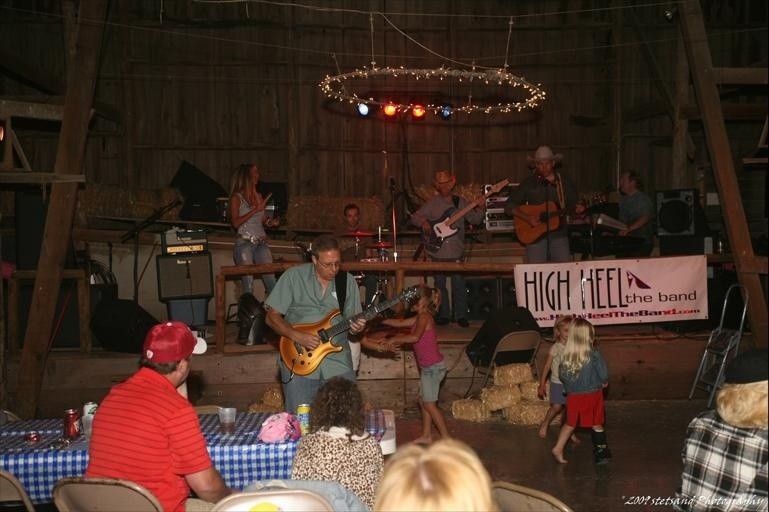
xmin=316 ymin=259 xmax=340 ymax=268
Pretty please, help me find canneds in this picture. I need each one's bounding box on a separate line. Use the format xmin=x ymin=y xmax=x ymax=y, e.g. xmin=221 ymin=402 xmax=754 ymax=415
xmin=26 ymin=431 xmax=40 ymax=442
xmin=83 ymin=402 xmax=98 ymax=419
xmin=296 ymin=404 xmax=311 ymax=436
xmin=65 ymin=409 xmax=81 ymax=441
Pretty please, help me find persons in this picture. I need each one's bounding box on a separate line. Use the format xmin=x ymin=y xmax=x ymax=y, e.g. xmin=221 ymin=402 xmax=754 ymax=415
xmin=265 ymin=235 xmax=366 ymax=412
xmin=374 ymin=434 xmax=499 ymax=511
xmin=348 ymin=335 xmax=398 ymax=378
xmin=505 ymin=146 xmax=585 ymax=263
xmin=230 ymin=164 xmax=281 ymax=297
xmin=537 ymin=316 xmax=581 ymax=442
xmin=375 ymin=284 xmax=452 ymax=443
xmin=413 ymin=169 xmax=486 ymax=327
xmin=84 ymin=320 xmax=240 ymax=511
xmin=602 ymin=170 xmax=656 ymax=257
xmin=292 ymin=376 xmax=383 ymax=510
xmin=676 ymin=349 xmax=769 ymax=511
xmin=553 ymin=318 xmax=612 ymax=466
xmin=334 ymin=202 xmax=378 ymax=307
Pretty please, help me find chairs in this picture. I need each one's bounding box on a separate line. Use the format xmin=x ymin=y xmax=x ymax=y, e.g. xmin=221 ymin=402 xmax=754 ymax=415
xmin=490 ymin=480 xmax=573 ymax=512
xmin=476 ymin=330 xmax=541 ymax=419
xmin=51 ymin=473 xmax=163 ymax=511
xmin=0 ymin=469 xmax=36 ymax=511
xmin=212 ymin=488 xmax=336 ymax=512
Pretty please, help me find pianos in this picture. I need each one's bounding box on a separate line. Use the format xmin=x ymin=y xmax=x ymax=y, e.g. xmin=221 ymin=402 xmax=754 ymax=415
xmin=569 ymin=209 xmax=629 ymax=232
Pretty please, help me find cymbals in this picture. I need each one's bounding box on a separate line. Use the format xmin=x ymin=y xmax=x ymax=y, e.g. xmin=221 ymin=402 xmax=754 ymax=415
xmin=339 ymin=230 xmax=375 ymax=237
xmin=366 ymin=241 xmax=394 ymax=248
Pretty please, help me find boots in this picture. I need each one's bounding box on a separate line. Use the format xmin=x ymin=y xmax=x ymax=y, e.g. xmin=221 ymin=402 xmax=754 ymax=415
xmin=591 ymin=431 xmax=613 ymax=465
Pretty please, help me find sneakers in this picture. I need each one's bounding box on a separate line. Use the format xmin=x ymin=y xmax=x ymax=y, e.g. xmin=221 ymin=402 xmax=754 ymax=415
xmin=458 ymin=317 xmax=470 ymax=328
xmin=434 ymin=317 xmax=451 ymax=326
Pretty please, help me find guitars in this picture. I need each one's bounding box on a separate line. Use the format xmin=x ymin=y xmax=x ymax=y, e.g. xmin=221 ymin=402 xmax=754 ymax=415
xmin=512 ymin=187 xmax=609 ymax=245
xmin=419 ymin=177 xmax=509 ymax=253
xmin=279 ymin=287 xmax=420 ymax=376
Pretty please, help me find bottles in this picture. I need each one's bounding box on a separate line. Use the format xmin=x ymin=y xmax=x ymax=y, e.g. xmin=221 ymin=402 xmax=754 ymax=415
xmin=715 ymin=228 xmax=725 ymax=257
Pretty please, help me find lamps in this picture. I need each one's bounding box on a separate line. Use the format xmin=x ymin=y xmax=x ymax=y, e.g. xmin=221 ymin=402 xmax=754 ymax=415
xmin=354 ymin=99 xmax=457 ymax=121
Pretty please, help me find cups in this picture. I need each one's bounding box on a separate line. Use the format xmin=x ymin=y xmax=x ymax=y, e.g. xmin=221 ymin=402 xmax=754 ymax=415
xmin=217 ymin=407 xmax=237 ymax=434
xmin=81 ymin=415 xmax=94 ymax=446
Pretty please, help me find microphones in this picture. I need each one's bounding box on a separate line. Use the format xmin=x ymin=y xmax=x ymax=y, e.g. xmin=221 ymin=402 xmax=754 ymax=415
xmin=160 ymin=196 xmax=183 ymax=215
xmin=389 ymin=177 xmax=396 ymax=194
xmin=536 ymin=175 xmax=557 ymax=188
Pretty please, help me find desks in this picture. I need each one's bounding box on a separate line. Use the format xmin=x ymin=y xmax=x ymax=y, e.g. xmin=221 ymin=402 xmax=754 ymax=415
xmin=0 ymin=409 xmax=396 ymax=512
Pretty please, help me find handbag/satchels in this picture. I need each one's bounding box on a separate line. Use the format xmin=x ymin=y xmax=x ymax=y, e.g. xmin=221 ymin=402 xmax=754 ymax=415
xmin=256 ymin=411 xmax=302 ymax=446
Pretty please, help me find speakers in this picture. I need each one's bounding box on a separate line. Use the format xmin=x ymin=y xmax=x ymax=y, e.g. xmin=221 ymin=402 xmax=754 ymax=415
xmin=466 ymin=307 xmax=539 ymax=367
xmin=16 ymin=187 xmax=74 ymax=271
xmin=90 ymin=300 xmax=162 ymax=353
xmin=500 ymin=275 xmax=518 ymax=307
xmin=464 ymin=275 xmax=499 ymax=319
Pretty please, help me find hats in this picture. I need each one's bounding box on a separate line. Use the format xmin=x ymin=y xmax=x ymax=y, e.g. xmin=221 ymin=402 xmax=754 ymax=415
xmin=143 ymin=321 xmax=207 ymax=365
xmin=431 ymin=171 xmax=456 ymax=189
xmin=528 ymin=145 xmax=563 ymax=162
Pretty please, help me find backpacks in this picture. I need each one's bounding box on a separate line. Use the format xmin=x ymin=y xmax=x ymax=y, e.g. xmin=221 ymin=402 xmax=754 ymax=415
xmin=236 ymin=293 xmax=266 ymax=346
